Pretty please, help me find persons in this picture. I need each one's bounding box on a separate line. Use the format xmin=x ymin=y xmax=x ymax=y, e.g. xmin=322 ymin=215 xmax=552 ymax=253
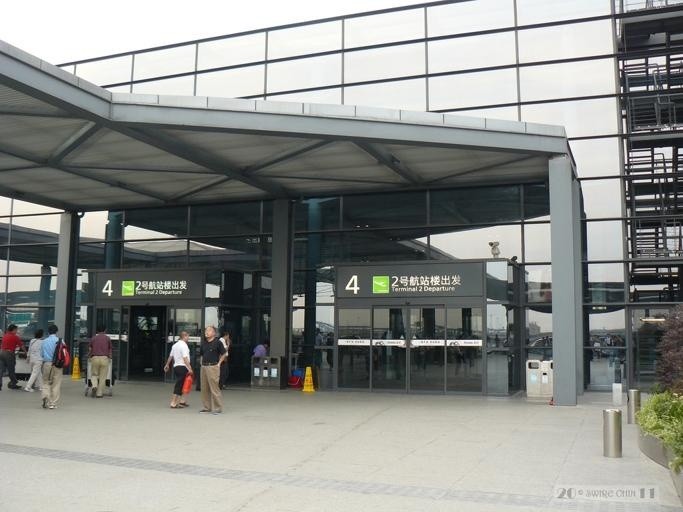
xmin=0 ymin=323 xmax=27 ymax=389
xmin=163 ymin=332 xmax=194 ymax=409
xmin=198 ymin=325 xmax=227 ymax=411
xmin=315 ymin=327 xmax=630 ymax=376
xmin=88 ymin=324 xmax=112 ymax=397
xmin=22 ymin=329 xmax=46 ymax=391
xmin=38 ymin=325 xmax=64 ymax=411
xmin=217 ymin=332 xmax=230 ymax=390
xmin=253 ymin=342 xmax=268 ymax=358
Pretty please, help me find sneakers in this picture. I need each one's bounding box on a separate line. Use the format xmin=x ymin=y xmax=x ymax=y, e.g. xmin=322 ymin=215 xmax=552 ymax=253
xmin=91 ymin=387 xmax=103 ymax=398
xmin=49 ymin=405 xmax=58 ymax=409
xmin=42 ymin=398 xmax=47 ymax=407
xmin=24 ymin=386 xmax=34 ymax=392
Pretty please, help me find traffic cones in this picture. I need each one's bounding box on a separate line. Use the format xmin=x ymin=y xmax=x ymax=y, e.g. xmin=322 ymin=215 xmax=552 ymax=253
xmin=299 ymin=367 xmax=315 ymax=393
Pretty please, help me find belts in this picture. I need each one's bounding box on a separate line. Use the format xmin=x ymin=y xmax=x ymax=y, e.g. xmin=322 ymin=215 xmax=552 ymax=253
xmin=202 ymin=362 xmax=217 ymax=365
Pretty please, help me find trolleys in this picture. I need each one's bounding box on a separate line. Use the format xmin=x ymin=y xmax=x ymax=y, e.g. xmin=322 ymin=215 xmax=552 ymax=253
xmin=6 ymin=348 xmax=38 ymax=389
xmin=82 ymin=351 xmax=115 ymax=397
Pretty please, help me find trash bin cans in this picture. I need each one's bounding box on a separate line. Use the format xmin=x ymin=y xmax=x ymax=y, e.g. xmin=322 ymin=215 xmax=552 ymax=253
xmin=526 ymin=359 xmax=553 ymax=398
xmin=603 ymin=409 xmax=622 ymax=459
xmin=627 ymin=389 xmax=641 ymax=424
xmin=251 ymin=355 xmax=288 ymax=390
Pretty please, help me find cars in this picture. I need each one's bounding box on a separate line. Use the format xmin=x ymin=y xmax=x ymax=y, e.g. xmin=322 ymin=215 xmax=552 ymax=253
xmin=526 ymin=337 xmax=552 ymax=353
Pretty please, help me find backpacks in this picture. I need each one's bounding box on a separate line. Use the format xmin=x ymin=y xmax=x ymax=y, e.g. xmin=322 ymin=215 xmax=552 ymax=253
xmin=52 ymin=338 xmax=70 ymax=368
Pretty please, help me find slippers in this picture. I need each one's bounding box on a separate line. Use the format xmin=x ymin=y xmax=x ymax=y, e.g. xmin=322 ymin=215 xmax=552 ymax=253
xmin=179 ymin=402 xmax=189 ymax=407
xmin=171 ymin=404 xmax=183 ymax=408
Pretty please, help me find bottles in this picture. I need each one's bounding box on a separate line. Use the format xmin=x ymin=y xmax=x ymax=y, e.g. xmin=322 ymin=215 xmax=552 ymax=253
xmin=633 ymin=288 xmax=638 ymax=301
